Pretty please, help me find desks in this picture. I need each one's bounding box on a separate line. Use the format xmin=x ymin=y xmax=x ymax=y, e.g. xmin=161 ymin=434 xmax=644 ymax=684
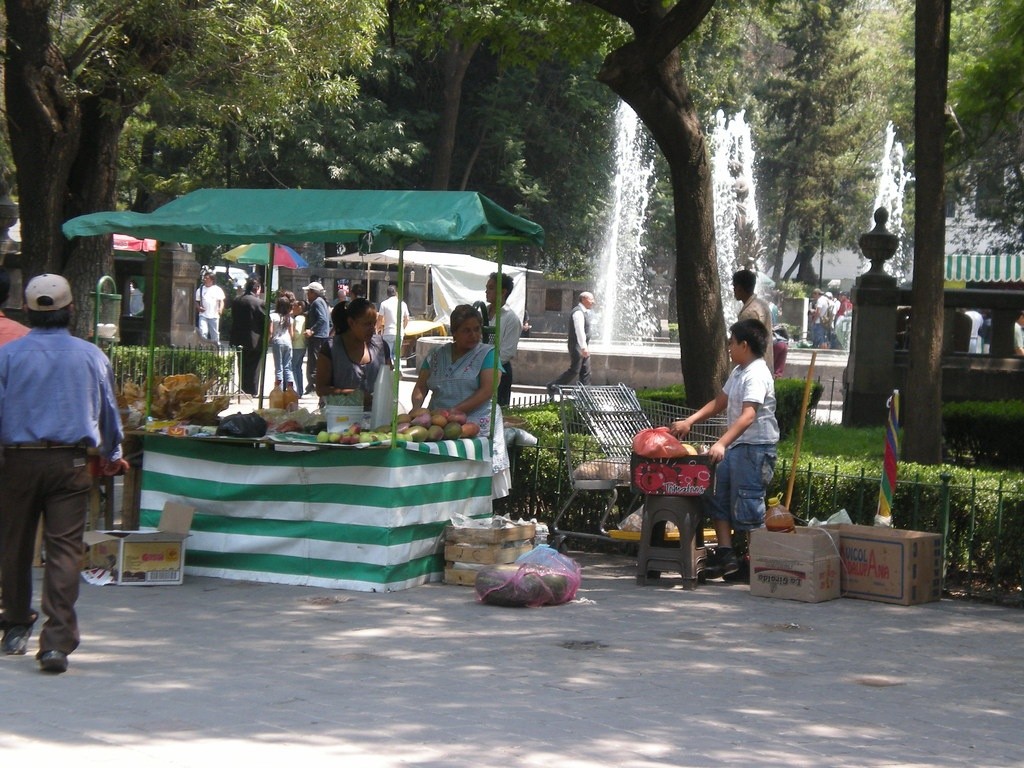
xmin=123 ymin=427 xmax=493 ymax=593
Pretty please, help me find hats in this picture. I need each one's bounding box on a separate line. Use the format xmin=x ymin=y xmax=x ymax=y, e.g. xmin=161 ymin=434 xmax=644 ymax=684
xmin=24 ymin=273 xmax=73 ymax=311
xmin=813 ymin=288 xmax=824 ymax=295
xmin=301 ymin=282 xmax=325 ymax=293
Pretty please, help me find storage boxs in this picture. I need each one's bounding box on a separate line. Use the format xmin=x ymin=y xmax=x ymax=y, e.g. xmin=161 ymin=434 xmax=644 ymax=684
xmin=819 ymin=522 xmax=943 ymax=605
xmin=749 ymin=526 xmax=841 ymax=604
xmin=630 ymin=452 xmax=717 ymax=496
xmin=81 ymin=500 xmax=196 ymax=586
xmin=443 ymin=523 xmax=536 ymax=586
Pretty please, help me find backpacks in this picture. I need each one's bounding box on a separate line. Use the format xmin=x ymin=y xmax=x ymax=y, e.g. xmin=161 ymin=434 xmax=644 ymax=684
xmin=820 ymin=304 xmax=834 ymax=326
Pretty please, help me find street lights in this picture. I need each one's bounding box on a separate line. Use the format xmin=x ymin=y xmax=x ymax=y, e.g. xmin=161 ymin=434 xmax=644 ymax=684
xmin=819 ymin=186 xmax=868 ymax=284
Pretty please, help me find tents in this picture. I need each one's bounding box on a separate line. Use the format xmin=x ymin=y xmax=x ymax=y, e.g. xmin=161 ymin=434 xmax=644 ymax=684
xmin=328 ymin=252 xmax=527 ymax=330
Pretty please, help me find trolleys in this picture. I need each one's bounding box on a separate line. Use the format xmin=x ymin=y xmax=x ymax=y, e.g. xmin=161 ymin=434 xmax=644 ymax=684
xmin=551 ymin=381 xmax=728 ymax=554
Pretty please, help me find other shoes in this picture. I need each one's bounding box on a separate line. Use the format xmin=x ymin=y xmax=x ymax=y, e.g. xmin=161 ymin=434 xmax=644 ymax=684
xmin=0 ymin=624 xmax=33 ymax=655
xmin=546 ymin=381 xmax=556 ymax=401
xmin=39 ymin=650 xmax=69 ymax=671
xmin=704 ymin=547 xmax=744 ymax=580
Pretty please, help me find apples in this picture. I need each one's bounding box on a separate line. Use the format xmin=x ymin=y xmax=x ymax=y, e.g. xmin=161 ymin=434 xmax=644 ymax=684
xmin=316 ymin=423 xmax=407 ymax=447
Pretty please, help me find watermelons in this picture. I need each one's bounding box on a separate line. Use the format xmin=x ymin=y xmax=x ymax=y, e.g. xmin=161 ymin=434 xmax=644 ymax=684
xmin=473 ymin=566 xmax=567 ymax=607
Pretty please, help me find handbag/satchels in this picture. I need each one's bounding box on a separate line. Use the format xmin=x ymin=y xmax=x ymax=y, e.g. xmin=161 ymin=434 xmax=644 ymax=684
xmin=631 ymin=426 xmax=689 ymax=460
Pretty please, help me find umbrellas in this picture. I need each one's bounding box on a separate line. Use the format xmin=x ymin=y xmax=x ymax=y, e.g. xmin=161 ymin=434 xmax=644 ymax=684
xmin=225 ymin=242 xmax=309 ymax=298
xmin=876 ymin=389 xmax=902 ymax=526
xmin=113 ymin=235 xmax=157 ymax=254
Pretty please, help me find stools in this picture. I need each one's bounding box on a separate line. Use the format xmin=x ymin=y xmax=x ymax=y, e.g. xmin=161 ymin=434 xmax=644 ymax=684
xmin=636 ymin=496 xmax=716 ymax=590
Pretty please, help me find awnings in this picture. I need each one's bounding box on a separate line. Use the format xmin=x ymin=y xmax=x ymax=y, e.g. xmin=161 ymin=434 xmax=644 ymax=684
xmin=62 ymin=188 xmax=545 ymax=253
xmin=905 ymin=252 xmax=1024 ymax=287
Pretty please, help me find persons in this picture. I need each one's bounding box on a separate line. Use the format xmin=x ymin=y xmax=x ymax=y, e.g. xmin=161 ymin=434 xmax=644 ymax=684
xmin=965 ymin=309 xmax=993 ymax=354
xmin=195 ymin=274 xmax=224 ymax=349
xmin=731 ymin=270 xmax=774 ymax=382
xmin=377 ymin=287 xmax=409 ymax=378
xmin=270 ymin=280 xmax=349 ymax=399
xmin=810 ymin=289 xmax=852 ymax=349
xmin=772 ymin=330 xmax=788 ymax=380
xmin=228 ymin=276 xmax=267 ymax=396
xmin=317 ymin=300 xmax=393 ymax=414
xmin=480 ymin=272 xmax=520 ymax=407
xmin=1014 ymin=311 xmax=1024 ymax=356
xmin=668 ymin=323 xmax=778 ymax=586
xmin=0 ymin=272 xmax=130 ymax=672
xmin=409 ymin=305 xmax=511 ymax=501
xmin=547 ymin=291 xmax=594 ymax=402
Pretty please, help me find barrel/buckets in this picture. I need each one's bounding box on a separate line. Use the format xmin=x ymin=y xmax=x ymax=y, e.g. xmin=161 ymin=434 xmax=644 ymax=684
xmin=325 ymin=405 xmax=365 ymax=433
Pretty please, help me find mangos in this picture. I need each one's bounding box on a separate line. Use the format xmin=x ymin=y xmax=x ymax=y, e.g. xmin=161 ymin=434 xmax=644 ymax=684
xmin=376 ymin=406 xmax=480 ymax=443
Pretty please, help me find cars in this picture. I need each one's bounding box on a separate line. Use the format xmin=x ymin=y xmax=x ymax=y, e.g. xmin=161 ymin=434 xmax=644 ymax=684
xmin=200 ymin=265 xmax=248 ymax=291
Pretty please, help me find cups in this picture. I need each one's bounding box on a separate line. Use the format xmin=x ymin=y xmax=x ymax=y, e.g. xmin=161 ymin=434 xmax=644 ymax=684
xmin=369 ymin=364 xmax=393 ymax=431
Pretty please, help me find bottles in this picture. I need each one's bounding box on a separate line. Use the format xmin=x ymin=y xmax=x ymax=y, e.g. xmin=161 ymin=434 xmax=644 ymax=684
xmin=284 ymin=382 xmax=298 ymax=410
xmin=269 ymin=381 xmax=284 ymax=409
xmin=764 ymin=497 xmax=797 ymax=535
